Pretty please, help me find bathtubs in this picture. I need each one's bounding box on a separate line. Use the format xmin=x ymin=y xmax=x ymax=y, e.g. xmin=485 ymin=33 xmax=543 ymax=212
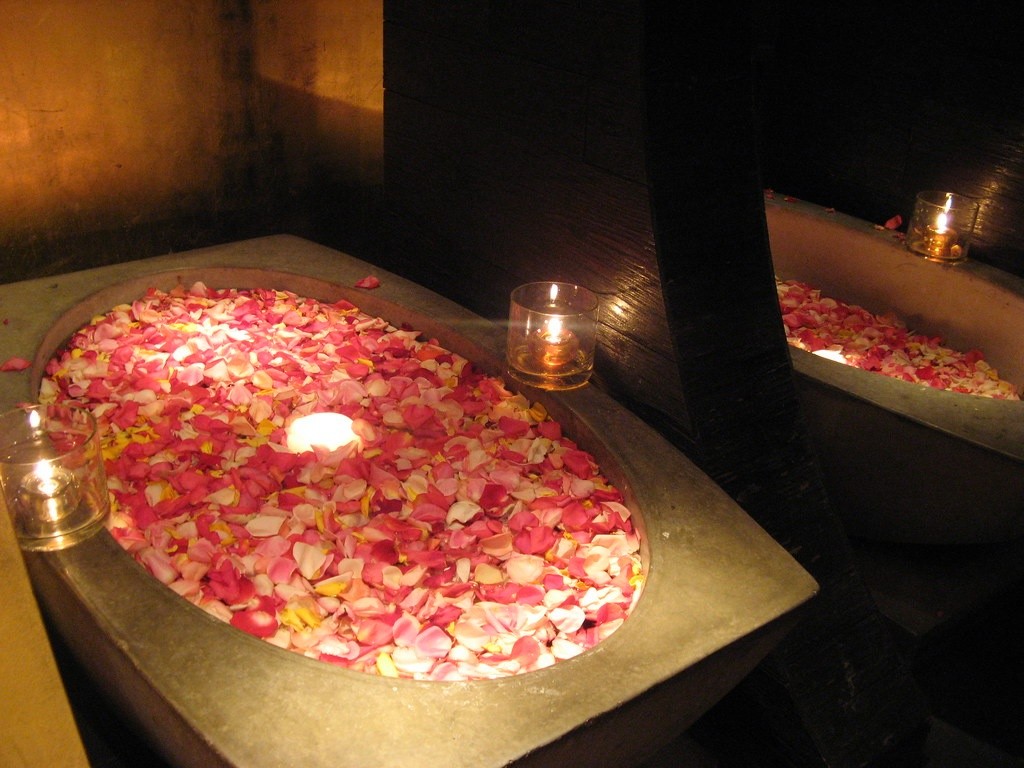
xmin=1 ymin=234 xmax=823 ymax=768
xmin=762 ymin=188 xmax=1024 ymax=547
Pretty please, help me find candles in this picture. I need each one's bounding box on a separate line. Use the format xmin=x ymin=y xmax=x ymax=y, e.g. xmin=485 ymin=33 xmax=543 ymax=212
xmin=918 ymin=212 xmax=958 ymax=256
xmin=526 ymin=316 xmax=578 ymax=368
xmin=16 ymin=458 xmax=80 ymax=523
